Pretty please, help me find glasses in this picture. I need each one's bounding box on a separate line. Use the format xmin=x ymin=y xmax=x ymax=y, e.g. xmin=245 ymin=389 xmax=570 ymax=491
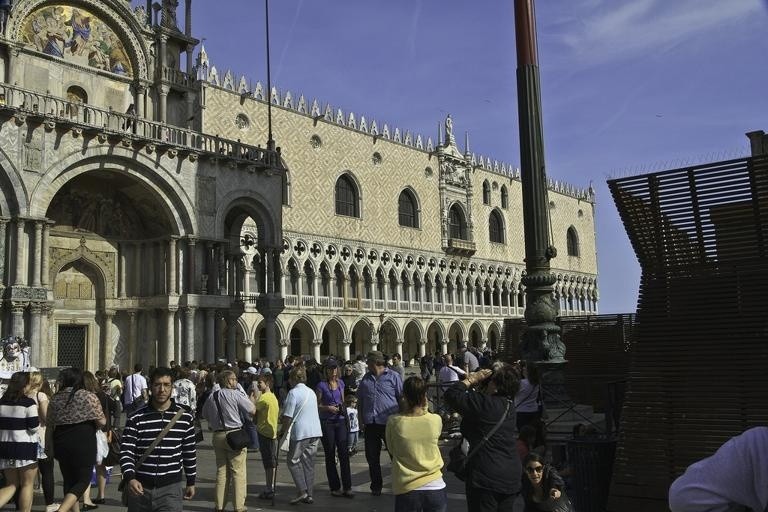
xmin=524 ymin=465 xmax=543 ymax=475
xmin=152 ymin=384 xmax=171 ymax=390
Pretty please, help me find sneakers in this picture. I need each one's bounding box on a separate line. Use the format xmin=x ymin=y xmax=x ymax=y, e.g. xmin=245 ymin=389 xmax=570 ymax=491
xmin=258 ymin=490 xmax=274 ymax=501
xmin=329 ymin=487 xmax=356 ymax=499
xmin=288 ymin=491 xmax=314 ymax=506
xmin=371 ymin=487 xmax=381 ymax=498
xmin=45 ymin=503 xmax=62 ymax=512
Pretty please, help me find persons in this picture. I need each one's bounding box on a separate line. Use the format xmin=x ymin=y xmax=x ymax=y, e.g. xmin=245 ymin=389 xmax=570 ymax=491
xmin=667 ymin=424 xmax=768 ymax=511
xmin=445 ymin=113 xmax=453 ymax=135
xmin=122 ymin=102 xmax=140 ymax=134
xmin=26 ymin=7 xmax=132 ymax=77
xmin=1 ymin=344 xmax=576 ymax=512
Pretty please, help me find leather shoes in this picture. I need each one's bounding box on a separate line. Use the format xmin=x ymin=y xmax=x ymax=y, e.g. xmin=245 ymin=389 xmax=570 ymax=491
xmin=83 ymin=502 xmax=99 ymax=511
xmin=90 ymin=498 xmax=105 ymax=504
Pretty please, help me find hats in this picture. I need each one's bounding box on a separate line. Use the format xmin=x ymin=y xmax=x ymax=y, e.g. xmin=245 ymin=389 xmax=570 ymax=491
xmin=321 ymin=358 xmax=337 ymax=368
xmin=242 ymin=366 xmax=257 ymax=376
xmin=362 ymin=350 xmax=386 ymax=363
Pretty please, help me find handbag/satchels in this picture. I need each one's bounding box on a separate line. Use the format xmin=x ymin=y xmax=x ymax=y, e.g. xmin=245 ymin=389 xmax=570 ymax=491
xmin=101 ymin=427 xmax=125 ymax=467
xmin=444 ymin=447 xmax=473 ymax=483
xmin=117 ymin=474 xmax=130 ymax=508
xmin=278 ymin=425 xmax=293 ymax=453
xmin=225 ymin=429 xmax=251 ymax=452
